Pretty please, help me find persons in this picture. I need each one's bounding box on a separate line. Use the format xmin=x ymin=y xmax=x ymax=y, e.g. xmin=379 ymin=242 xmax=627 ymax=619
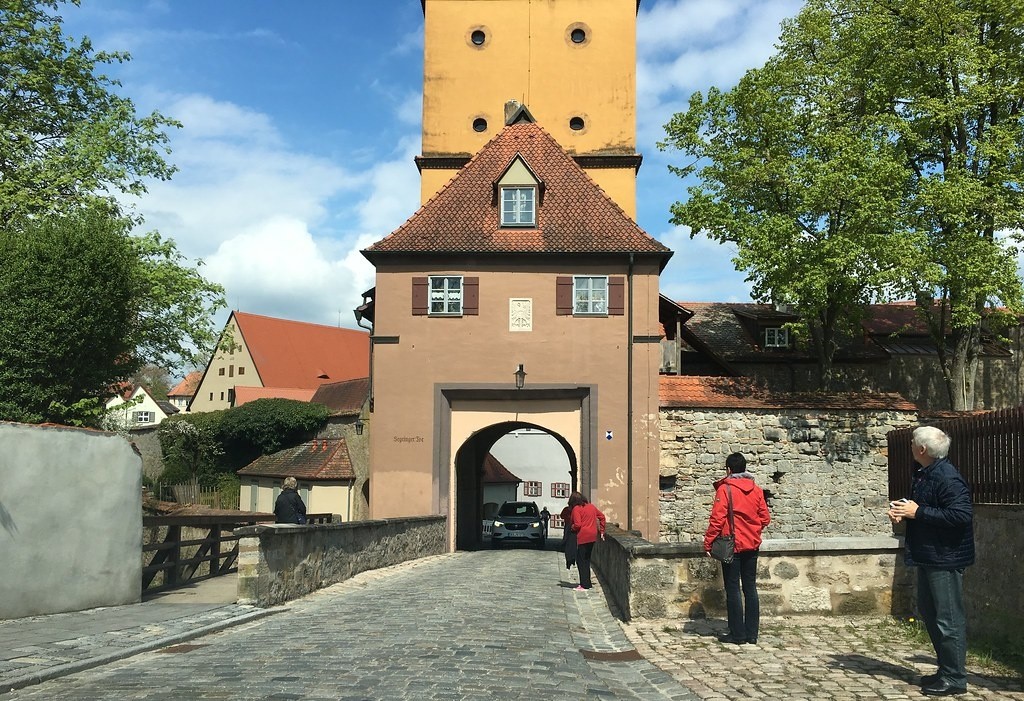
xmin=704 ymin=452 xmax=771 ymax=644
xmin=540 ymin=507 xmax=551 ymax=539
xmin=274 ymin=478 xmax=306 ymax=524
xmin=888 ymin=426 xmax=975 ymax=694
xmin=560 ymin=492 xmax=606 ymax=592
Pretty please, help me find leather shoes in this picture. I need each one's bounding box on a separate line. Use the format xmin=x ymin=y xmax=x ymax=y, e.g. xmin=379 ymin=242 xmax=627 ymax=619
xmin=923 ymin=679 xmax=967 ymax=696
xmin=921 ymin=673 xmax=940 ymax=686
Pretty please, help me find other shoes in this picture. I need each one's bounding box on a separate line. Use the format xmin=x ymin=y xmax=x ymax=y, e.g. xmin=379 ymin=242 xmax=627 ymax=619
xmin=746 ymin=638 xmax=756 ymax=644
xmin=573 ymin=585 xmax=591 ymax=591
xmin=718 ymin=635 xmax=746 ymax=644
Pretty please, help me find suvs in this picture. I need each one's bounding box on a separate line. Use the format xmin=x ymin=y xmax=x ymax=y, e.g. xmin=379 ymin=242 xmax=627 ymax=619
xmin=491 ymin=499 xmax=544 ymax=549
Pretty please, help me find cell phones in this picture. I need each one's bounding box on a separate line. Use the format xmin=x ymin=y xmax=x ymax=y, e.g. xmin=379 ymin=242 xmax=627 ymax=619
xmin=889 ymin=498 xmax=910 ymax=507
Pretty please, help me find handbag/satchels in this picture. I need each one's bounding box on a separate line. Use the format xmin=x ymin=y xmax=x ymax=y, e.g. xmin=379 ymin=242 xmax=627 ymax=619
xmin=295 ymin=513 xmax=305 ymax=523
xmin=710 ymin=532 xmax=735 ymax=563
